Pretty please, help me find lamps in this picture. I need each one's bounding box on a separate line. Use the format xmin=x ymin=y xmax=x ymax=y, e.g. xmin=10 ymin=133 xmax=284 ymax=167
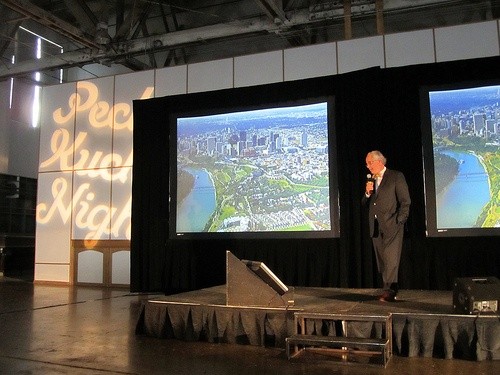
xmin=94 ymin=22 xmax=111 ymax=44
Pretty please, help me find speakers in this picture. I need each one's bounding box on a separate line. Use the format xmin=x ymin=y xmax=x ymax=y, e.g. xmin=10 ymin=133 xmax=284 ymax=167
xmin=453 ymin=277 xmax=500 ymax=316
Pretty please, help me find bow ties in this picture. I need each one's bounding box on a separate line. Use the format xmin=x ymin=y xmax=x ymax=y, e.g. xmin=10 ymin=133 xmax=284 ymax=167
xmin=372 ymin=173 xmax=381 ymax=179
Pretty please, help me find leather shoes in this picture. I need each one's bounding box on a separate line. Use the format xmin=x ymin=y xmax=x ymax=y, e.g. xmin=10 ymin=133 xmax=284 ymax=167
xmin=379 ymin=288 xmax=398 ymax=302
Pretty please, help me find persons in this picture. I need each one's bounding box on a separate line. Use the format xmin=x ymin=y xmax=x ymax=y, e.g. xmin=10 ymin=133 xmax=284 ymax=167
xmin=363 ymin=151 xmax=411 ymax=302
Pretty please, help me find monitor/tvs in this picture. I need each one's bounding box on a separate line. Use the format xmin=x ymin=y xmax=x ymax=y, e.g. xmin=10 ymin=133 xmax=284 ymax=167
xmin=242 ymin=259 xmax=288 ymax=295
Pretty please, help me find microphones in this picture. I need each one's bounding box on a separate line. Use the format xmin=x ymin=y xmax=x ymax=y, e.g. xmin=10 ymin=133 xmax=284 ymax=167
xmin=367 ymin=174 xmax=373 ymax=194
